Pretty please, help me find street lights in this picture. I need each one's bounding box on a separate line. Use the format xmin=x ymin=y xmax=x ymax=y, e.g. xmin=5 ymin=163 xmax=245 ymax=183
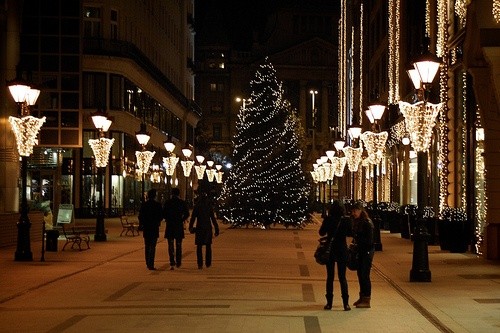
xmin=7 ymin=63 xmax=41 ymax=263
xmin=399 ymin=36 xmax=446 ymax=282
xmin=360 ymin=96 xmax=390 ymax=252
xmin=181 ymin=140 xmax=223 ymax=209
xmin=134 ymin=117 xmax=156 ymax=205
xmin=309 ymin=114 xmax=364 ymax=218
xmin=88 ymin=103 xmax=113 ymax=241
xmin=162 ymin=131 xmax=180 ymax=201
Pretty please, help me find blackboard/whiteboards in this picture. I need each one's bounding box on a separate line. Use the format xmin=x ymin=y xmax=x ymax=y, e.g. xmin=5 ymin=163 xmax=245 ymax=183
xmin=57 ymin=204 xmax=74 ymax=224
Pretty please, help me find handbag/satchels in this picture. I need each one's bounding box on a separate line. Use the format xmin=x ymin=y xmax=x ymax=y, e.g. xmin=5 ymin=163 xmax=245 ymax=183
xmin=314 ymin=237 xmax=331 ymax=265
xmin=345 ymin=233 xmax=359 ymax=271
xmin=138 ymin=222 xmax=145 ymax=231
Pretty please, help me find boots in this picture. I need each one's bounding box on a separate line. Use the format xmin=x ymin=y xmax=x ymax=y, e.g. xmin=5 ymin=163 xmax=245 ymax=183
xmin=342 ymin=295 xmax=351 ymax=311
xmin=324 ymin=295 xmax=333 ymax=309
xmin=356 ymin=296 xmax=370 ymax=308
xmin=354 ymin=292 xmax=362 ymax=306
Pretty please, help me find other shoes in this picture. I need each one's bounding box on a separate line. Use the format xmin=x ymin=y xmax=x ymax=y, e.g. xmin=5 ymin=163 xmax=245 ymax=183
xmin=198 ymin=263 xmax=203 ymax=270
xmin=176 ymin=262 xmax=181 ymax=267
xmin=147 ymin=263 xmax=150 ymax=268
xmin=170 ymin=261 xmax=175 ymax=267
xmin=149 ymin=265 xmax=156 ymax=270
xmin=206 ymin=263 xmax=211 ymax=267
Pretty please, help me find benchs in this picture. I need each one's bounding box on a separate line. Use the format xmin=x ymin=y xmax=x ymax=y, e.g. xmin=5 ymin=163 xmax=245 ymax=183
xmin=120 ymin=217 xmax=140 ymax=236
xmin=62 ymin=223 xmax=90 ymax=251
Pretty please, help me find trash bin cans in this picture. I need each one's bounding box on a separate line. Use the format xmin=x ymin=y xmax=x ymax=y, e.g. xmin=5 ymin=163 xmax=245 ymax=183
xmin=46 ymin=230 xmax=59 ymax=252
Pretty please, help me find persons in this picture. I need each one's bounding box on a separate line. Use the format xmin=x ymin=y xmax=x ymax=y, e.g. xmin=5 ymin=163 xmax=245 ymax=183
xmin=163 ymin=188 xmax=190 ymax=272
xmin=189 ymin=193 xmax=219 ymax=269
xmin=138 ymin=190 xmax=163 ymax=271
xmin=319 ymin=200 xmax=351 ymax=311
xmin=348 ymin=204 xmax=375 ymax=310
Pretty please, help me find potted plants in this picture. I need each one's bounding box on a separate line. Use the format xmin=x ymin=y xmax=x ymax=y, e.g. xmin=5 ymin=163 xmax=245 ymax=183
xmin=365 ymin=200 xmax=470 ymax=253
